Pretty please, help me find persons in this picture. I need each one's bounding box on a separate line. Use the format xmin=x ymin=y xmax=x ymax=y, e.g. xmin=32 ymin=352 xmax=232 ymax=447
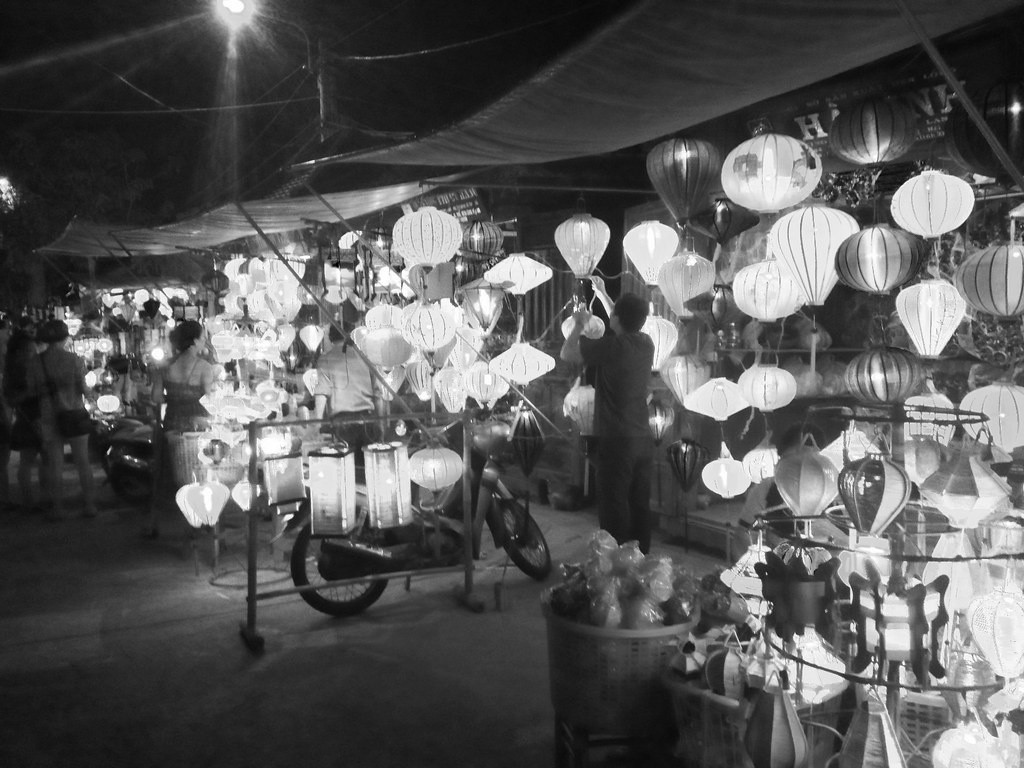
xmin=314 ymin=319 xmax=384 ymax=468
xmin=560 ymin=292 xmax=654 ymax=555
xmin=139 ymin=318 xmax=214 ymax=540
xmin=0 ymin=307 xmax=130 ymax=520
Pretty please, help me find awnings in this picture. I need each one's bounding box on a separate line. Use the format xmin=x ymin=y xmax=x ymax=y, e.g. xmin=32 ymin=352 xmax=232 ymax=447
xmin=27 ymin=1 xmax=1022 ymax=270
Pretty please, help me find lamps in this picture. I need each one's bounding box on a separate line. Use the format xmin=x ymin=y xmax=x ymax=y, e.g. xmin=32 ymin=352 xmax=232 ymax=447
xmin=733 ymin=261 xmax=804 ymax=322
xmin=483 ymin=252 xmax=553 ymax=295
xmin=701 ymin=441 xmax=750 ymax=498
xmin=61 ymin=229 xmax=355 ymax=536
xmin=659 ymin=352 xmax=710 ymax=408
xmin=646 ymin=135 xmax=721 ymax=224
xmin=743 ymin=430 xmax=778 ymax=484
xmin=639 ymin=314 xmax=679 ymax=376
xmin=488 ymin=342 xmax=556 ymax=386
xmin=352 ymin=200 xmax=509 ymax=532
xmin=739 ymin=363 xmax=797 ymax=412
xmin=645 ymin=397 xmax=674 ymax=445
xmin=684 ymin=376 xmax=751 ymax=421
xmin=622 ymin=220 xmax=679 ymax=288
xmin=562 ymin=384 xmax=598 ymax=436
xmin=554 ymin=214 xmax=611 ymax=280
xmin=770 ymin=88 xmax=1024 ymax=768
xmin=561 ymin=312 xmax=605 ymax=340
xmin=657 ymin=249 xmax=714 ymax=322
xmin=720 ymin=124 xmax=823 ymax=213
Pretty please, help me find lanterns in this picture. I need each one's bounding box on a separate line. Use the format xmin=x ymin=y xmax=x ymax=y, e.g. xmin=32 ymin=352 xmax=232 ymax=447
xmin=73 ymin=206 xmax=554 ymax=528
xmin=556 ymin=62 xmax=1024 ymax=768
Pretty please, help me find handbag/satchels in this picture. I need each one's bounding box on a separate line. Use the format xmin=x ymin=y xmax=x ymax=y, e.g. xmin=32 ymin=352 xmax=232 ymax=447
xmin=57 ymin=409 xmax=98 ymax=440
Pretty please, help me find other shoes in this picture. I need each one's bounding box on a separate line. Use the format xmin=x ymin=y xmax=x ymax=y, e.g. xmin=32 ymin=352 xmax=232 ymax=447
xmin=22 ymin=496 xmax=37 ymax=509
xmin=82 ymin=505 xmax=99 ymax=516
xmin=46 ymin=508 xmax=67 ymax=521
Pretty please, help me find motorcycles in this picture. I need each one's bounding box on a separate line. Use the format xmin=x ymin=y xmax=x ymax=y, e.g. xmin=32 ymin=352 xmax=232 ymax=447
xmin=289 ymin=402 xmax=553 ymax=614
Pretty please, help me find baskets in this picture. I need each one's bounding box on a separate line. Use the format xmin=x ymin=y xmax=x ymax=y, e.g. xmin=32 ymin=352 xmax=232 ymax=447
xmin=539 ymin=580 xmax=703 ymax=734
